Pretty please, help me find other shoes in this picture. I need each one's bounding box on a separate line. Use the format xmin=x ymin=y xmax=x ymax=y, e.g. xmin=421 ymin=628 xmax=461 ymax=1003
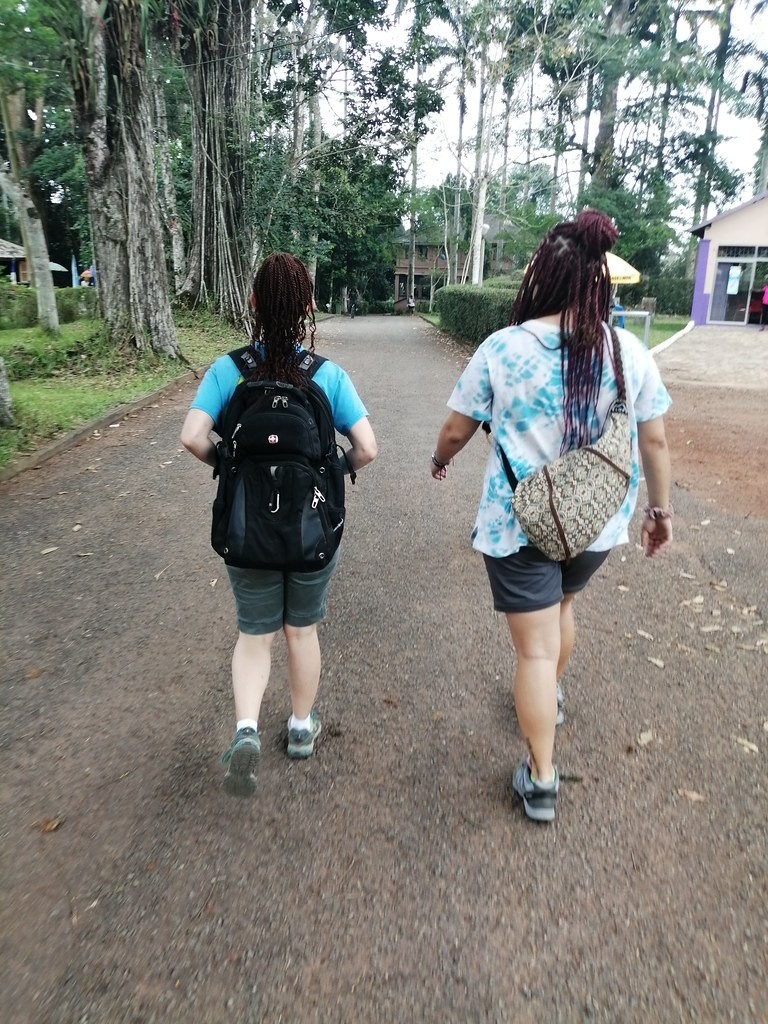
xmin=759 ymin=325 xmax=765 ymax=331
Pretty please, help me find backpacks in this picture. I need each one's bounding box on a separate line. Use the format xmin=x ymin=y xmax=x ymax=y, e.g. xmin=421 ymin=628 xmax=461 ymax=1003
xmin=212 ymin=346 xmax=357 ymax=574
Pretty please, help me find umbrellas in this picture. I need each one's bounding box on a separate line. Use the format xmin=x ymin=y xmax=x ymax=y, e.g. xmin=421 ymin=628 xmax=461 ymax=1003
xmin=522 ymin=249 xmax=641 ymax=284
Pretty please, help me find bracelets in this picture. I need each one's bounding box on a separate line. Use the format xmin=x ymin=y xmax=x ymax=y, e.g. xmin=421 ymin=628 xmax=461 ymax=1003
xmin=644 ymin=505 xmax=676 ymax=519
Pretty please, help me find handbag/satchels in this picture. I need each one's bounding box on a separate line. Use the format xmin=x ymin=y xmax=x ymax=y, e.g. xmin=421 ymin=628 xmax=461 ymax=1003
xmin=510 ymin=400 xmax=633 ymax=567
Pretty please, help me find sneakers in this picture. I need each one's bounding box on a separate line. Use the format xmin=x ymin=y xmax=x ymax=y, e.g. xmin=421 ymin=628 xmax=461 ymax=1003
xmin=287 ymin=709 xmax=321 ymax=757
xmin=555 ymin=682 xmax=565 ymax=725
xmin=513 ymin=761 xmax=559 ymax=821
xmin=221 ymin=726 xmax=261 ymax=799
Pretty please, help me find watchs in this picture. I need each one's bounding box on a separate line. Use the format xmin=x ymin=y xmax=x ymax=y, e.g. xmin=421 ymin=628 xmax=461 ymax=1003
xmin=431 ymin=450 xmax=448 ymax=468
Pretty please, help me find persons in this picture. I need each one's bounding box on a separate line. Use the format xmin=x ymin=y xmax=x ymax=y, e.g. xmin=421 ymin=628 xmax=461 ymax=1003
xmin=180 ymin=250 xmax=377 ymax=802
xmin=759 ymin=285 xmax=768 ymax=314
xmin=408 ymin=295 xmax=416 ymax=315
xmin=427 ymin=208 xmax=673 ymax=823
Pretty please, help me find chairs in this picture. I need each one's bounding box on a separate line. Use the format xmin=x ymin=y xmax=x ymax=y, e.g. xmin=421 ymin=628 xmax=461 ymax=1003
xmin=731 ymin=290 xmax=765 ymax=325
